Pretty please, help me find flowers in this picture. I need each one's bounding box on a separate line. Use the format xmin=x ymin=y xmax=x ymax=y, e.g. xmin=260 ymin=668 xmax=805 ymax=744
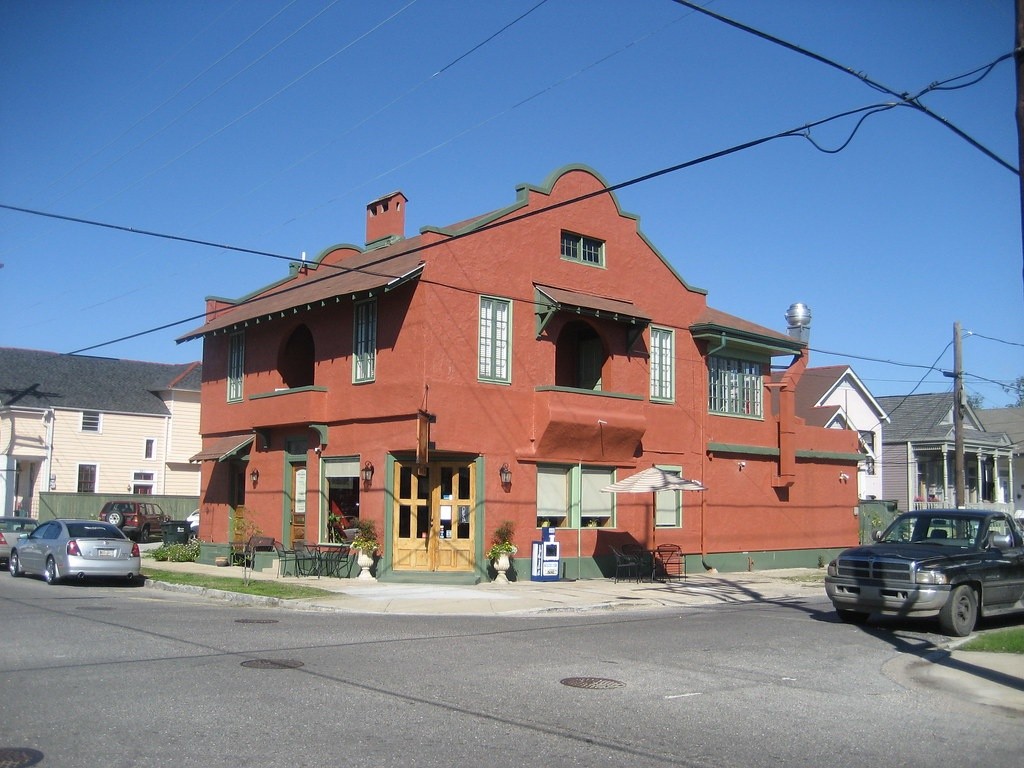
xmin=352 ymin=536 xmax=383 ymax=557
xmin=485 ymin=539 xmax=512 ymax=560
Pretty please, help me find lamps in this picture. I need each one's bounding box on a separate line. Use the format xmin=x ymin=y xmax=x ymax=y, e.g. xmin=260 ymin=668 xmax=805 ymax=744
xmin=501 ymin=463 xmax=512 ymax=485
xmin=250 ymin=468 xmax=259 ymax=481
xmin=361 ymin=461 xmax=374 ymax=481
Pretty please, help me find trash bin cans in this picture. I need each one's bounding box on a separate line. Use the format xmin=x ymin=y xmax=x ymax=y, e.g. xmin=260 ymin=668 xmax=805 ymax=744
xmin=160 ymin=520 xmax=193 ymax=546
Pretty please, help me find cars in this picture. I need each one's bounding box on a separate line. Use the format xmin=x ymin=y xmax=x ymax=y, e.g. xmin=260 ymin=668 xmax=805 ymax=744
xmin=185 ymin=508 xmax=199 ymax=532
xmin=0 ymin=516 xmax=43 ymax=563
xmin=9 ymin=518 xmax=141 ymax=585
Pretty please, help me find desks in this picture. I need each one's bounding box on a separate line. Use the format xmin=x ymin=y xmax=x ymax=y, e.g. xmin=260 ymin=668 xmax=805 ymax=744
xmin=635 ymin=550 xmax=677 ymax=583
xmin=303 ymin=544 xmax=350 ymax=578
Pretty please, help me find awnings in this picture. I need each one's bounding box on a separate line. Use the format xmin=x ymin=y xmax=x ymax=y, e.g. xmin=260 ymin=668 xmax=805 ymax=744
xmin=193 ymin=434 xmax=254 ymax=463
xmin=174 ymin=263 xmax=424 ymax=345
xmin=689 ymin=323 xmax=809 ymax=356
xmin=535 ymin=284 xmax=651 ymax=356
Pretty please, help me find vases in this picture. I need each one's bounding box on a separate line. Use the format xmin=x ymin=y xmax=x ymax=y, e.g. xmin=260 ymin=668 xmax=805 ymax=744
xmin=489 ymin=551 xmax=512 ymax=585
xmin=357 ymin=546 xmax=377 ymax=582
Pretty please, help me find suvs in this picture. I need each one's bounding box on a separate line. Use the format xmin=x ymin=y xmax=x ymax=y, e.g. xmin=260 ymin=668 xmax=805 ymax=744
xmin=99 ymin=501 xmax=170 ymax=543
xmin=825 ymin=508 xmax=1024 ymax=638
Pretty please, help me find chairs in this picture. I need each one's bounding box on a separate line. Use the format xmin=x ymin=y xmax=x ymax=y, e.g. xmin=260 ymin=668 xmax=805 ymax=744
xmin=235 ymin=536 xmax=275 ymax=569
xmin=273 ymin=539 xmax=356 ymax=579
xmin=609 ymin=544 xmax=687 ymax=584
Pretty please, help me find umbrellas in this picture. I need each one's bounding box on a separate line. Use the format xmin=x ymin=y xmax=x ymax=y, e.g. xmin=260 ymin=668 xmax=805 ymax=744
xmin=600 ymin=465 xmax=708 ymax=582
